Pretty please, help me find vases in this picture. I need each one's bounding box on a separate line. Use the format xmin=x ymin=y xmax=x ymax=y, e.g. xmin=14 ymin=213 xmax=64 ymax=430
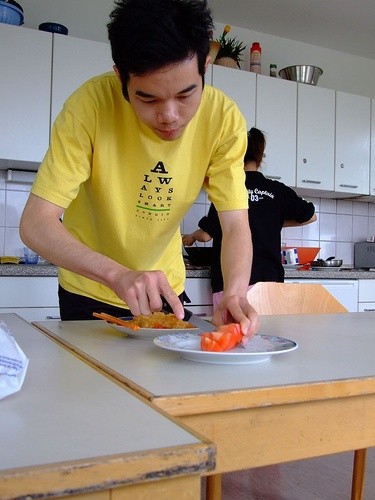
xmin=216 ymin=57 xmax=237 ymax=69
xmin=207 ymin=41 xmax=221 ymax=64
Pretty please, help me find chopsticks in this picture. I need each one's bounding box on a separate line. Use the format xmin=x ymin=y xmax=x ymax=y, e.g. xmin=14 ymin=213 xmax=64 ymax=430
xmin=93 ymin=312 xmax=141 ymax=331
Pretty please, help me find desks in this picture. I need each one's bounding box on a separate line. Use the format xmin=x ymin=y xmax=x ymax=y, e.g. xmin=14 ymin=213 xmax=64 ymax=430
xmin=31 ymin=312 xmax=374 ymax=500
xmin=0 ymin=312 xmax=217 ymax=500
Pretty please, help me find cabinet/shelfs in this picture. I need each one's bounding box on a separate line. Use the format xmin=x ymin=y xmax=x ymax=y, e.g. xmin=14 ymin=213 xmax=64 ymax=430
xmin=0 ymin=23 xmax=116 ymax=171
xmin=1 ymin=275 xmax=214 ymax=324
xmin=211 ymin=65 xmax=375 ymax=203
xmin=358 ymin=278 xmax=375 ymax=312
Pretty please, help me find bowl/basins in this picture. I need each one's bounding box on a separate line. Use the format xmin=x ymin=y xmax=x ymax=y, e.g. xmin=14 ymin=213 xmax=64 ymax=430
xmin=278 ymin=65 xmax=323 ymax=86
xmin=322 ymin=260 xmax=342 ymax=267
xmin=0 ymin=0 xmax=25 ymax=26
xmin=38 ymin=22 xmax=69 ymax=35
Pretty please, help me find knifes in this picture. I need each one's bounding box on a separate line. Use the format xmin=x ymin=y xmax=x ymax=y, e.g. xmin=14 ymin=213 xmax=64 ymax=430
xmin=161 ymin=297 xmax=246 ymax=348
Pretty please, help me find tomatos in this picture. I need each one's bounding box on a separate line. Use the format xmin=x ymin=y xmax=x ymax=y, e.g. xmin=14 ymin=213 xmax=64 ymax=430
xmin=201 ymin=323 xmax=242 ymax=352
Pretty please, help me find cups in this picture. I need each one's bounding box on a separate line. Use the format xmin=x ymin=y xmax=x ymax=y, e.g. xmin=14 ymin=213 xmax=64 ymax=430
xmin=283 ymin=248 xmax=298 ymax=265
xmin=24 ymin=247 xmax=39 ymax=265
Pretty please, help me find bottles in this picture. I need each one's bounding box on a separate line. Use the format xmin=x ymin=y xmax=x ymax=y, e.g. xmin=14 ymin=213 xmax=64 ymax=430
xmin=249 ymin=42 xmax=262 ymax=75
xmin=270 ymin=64 xmax=277 ymax=77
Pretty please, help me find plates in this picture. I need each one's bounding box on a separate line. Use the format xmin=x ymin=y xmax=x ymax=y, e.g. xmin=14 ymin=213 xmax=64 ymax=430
xmin=108 ymin=317 xmax=197 ymax=339
xmin=312 ymin=267 xmax=340 ymax=271
xmin=283 ymin=265 xmax=303 ymax=270
xmin=154 ymin=331 xmax=296 ymax=362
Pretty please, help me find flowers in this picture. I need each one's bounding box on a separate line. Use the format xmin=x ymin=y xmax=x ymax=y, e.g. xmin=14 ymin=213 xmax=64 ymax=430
xmin=213 ymin=25 xmax=246 ymax=70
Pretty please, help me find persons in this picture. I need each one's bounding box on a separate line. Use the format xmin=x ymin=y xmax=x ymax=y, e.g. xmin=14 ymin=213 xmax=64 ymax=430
xmin=18 ymin=0 xmax=260 ymax=348
xmin=182 ymin=128 xmax=317 ymax=312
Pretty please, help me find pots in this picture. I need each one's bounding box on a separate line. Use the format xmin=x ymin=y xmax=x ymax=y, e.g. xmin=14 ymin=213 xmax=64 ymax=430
xmin=184 ymin=241 xmax=213 ymax=266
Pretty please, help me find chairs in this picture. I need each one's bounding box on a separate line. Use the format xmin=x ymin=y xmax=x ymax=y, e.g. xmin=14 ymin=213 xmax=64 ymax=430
xmin=246 ymin=282 xmax=348 ymax=315
